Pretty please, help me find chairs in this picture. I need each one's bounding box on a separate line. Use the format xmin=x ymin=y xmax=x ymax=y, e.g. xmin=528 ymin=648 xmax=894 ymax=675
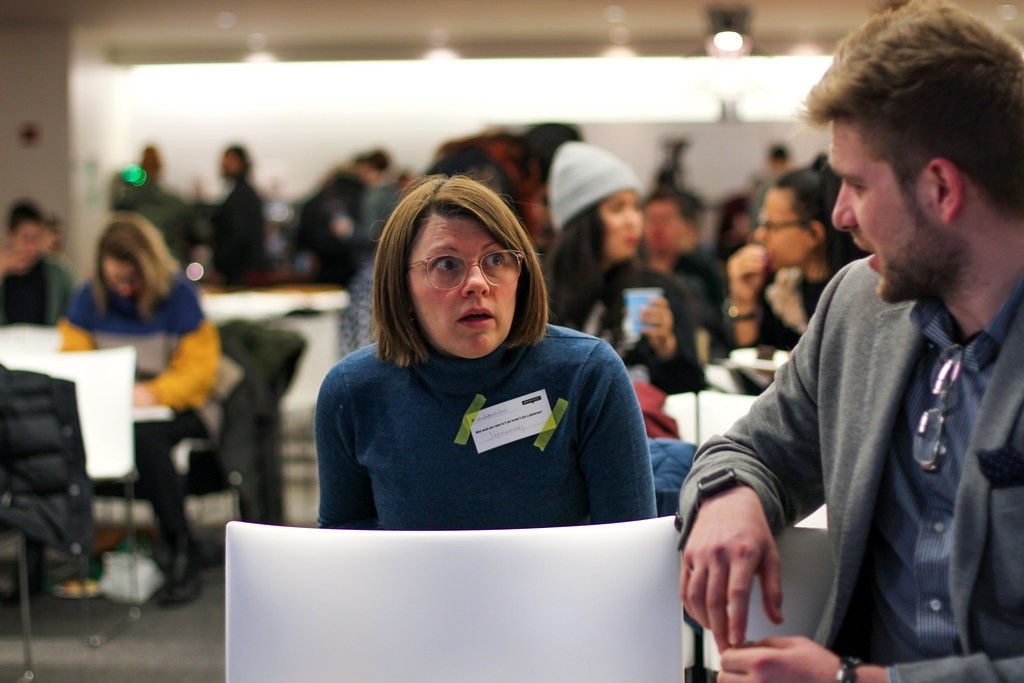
xmin=1 ymin=290 xmax=829 ymax=681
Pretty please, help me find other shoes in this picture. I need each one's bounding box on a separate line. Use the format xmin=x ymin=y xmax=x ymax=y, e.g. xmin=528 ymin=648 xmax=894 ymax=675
xmin=158 ymin=534 xmax=205 ymax=603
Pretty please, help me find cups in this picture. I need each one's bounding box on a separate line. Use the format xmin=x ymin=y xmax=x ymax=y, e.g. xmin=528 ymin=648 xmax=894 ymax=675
xmin=622 ymin=287 xmax=663 ymax=334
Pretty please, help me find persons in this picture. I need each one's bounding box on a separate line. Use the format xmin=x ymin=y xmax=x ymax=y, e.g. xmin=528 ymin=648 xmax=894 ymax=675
xmin=63 ymin=207 xmax=226 ymax=605
xmin=673 ymin=0 xmax=1024 ymax=683
xmin=1 ymin=199 xmax=77 ymax=336
xmin=318 ymin=168 xmax=659 ymax=533
xmin=118 ymin=123 xmax=868 ymax=395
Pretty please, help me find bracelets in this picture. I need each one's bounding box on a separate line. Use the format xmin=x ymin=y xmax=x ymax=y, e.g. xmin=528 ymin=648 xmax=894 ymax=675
xmin=837 ymin=654 xmax=864 ymax=682
xmin=730 ymin=311 xmax=763 ymax=323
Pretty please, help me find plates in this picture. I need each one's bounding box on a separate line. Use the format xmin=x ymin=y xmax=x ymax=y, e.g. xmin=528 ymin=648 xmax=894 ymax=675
xmin=729 ymin=346 xmax=792 ymax=370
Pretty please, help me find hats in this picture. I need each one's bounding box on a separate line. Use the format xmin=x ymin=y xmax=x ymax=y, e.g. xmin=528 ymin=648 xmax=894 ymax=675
xmin=548 ymin=140 xmax=645 ymax=230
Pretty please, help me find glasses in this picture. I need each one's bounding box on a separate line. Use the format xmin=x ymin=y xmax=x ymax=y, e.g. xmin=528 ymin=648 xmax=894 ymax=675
xmin=912 ymin=340 xmax=962 ymax=473
xmin=407 ymin=249 xmax=525 ymax=290
xmin=100 ymin=268 xmax=143 ymax=289
xmin=751 ymin=213 xmax=814 ymax=234
